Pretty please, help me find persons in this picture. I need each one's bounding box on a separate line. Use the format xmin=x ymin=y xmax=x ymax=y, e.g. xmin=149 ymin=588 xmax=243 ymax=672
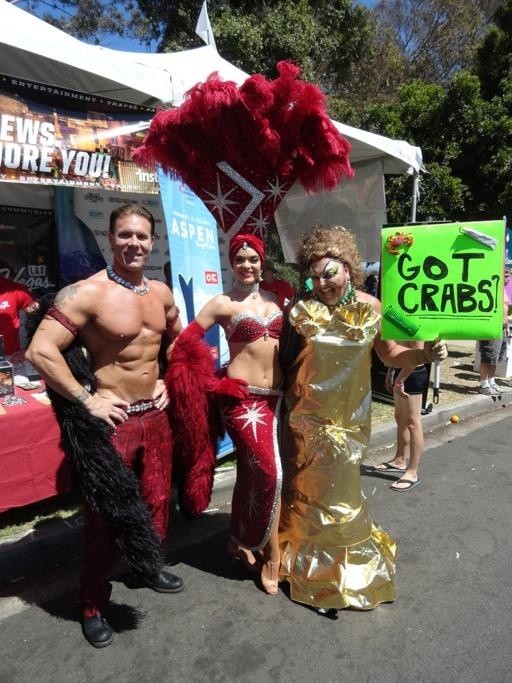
xmin=25 ymin=203 xmax=184 ymax=648
xmin=478 ymin=269 xmax=512 ymax=397
xmin=279 ymin=226 xmax=448 ymax=610
xmin=166 ymin=233 xmax=290 ymax=595
xmin=259 ymin=260 xmax=297 ymax=315
xmin=0 ymin=276 xmax=39 ymax=356
xmin=374 ymin=340 xmax=429 ymax=492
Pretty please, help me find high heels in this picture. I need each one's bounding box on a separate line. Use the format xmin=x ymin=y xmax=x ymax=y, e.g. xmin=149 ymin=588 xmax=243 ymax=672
xmin=227 ymin=542 xmax=258 ymax=572
xmin=261 ymin=566 xmax=279 ymax=595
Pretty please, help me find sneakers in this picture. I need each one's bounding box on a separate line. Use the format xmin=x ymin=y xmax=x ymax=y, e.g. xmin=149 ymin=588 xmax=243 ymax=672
xmin=477 ymin=385 xmax=503 ymax=395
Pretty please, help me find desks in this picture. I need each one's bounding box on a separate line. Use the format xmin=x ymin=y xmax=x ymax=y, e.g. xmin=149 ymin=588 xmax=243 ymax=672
xmin=0 ymin=352 xmax=63 ymax=516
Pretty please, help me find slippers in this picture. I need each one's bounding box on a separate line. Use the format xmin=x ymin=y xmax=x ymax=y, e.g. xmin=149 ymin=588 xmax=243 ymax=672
xmin=372 ymin=462 xmax=407 ymax=472
xmin=390 ymin=479 xmax=421 ymax=492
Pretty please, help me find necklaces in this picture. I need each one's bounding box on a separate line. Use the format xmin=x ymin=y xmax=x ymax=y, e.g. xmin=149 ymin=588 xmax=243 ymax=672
xmin=234 ymin=279 xmax=259 ymax=293
xmin=106 ymin=264 xmax=149 ymax=296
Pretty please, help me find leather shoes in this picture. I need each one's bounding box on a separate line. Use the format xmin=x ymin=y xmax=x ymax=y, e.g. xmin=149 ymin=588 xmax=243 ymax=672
xmin=82 ymin=606 xmax=113 ymax=648
xmin=123 ymin=570 xmax=184 ymax=593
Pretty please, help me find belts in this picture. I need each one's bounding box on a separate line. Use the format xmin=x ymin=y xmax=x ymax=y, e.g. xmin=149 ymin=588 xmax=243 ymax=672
xmin=127 ymin=399 xmax=159 ymax=412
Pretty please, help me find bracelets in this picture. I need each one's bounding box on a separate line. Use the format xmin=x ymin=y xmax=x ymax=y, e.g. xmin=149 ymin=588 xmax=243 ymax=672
xmin=412 ymin=349 xmax=419 ymax=365
